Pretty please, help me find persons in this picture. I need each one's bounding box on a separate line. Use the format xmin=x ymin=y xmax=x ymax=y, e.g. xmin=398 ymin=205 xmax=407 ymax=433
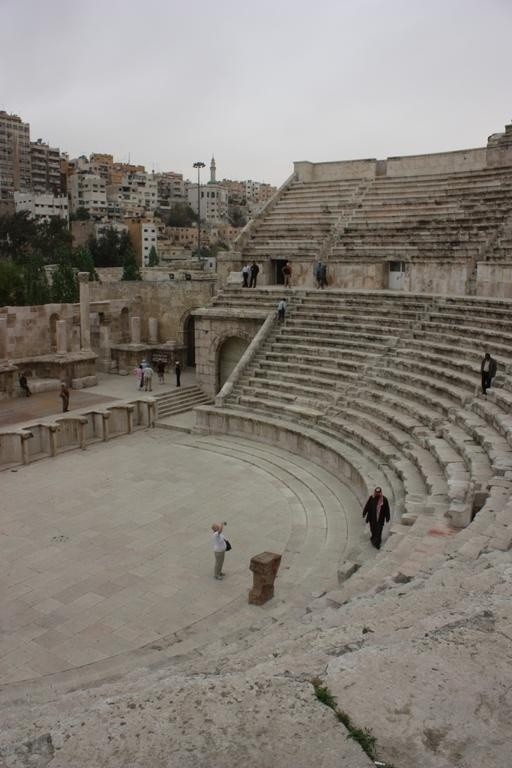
xmin=241 ymin=262 xmax=249 ymax=287
xmin=175 ymin=361 xmax=181 ymax=387
xmin=156 ymin=359 xmax=165 ymax=385
xmin=141 ymin=364 xmax=154 ymax=392
xmin=134 ymin=364 xmax=143 ymax=391
xmin=282 ymin=262 xmax=292 ymax=289
xmin=315 ymin=261 xmax=324 ymax=290
xmin=362 ymin=487 xmax=390 ymax=550
xmin=276 ymin=297 xmax=287 ymax=320
xmin=20 ymin=373 xmax=31 ymax=397
xmin=210 ymin=520 xmax=227 ymax=581
xmin=322 ymin=265 xmax=328 ymax=287
xmin=248 ymin=259 xmax=259 ymax=288
xmin=59 ymin=382 xmax=70 ymax=413
xmin=479 ymin=353 xmax=496 ymax=395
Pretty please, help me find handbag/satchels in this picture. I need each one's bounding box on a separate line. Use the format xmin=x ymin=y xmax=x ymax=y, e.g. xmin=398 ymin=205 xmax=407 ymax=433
xmin=225 ymin=540 xmax=231 ymax=551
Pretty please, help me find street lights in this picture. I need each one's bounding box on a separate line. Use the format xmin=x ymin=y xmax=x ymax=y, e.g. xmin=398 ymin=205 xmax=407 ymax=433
xmin=193 ymin=161 xmax=206 ymax=258
